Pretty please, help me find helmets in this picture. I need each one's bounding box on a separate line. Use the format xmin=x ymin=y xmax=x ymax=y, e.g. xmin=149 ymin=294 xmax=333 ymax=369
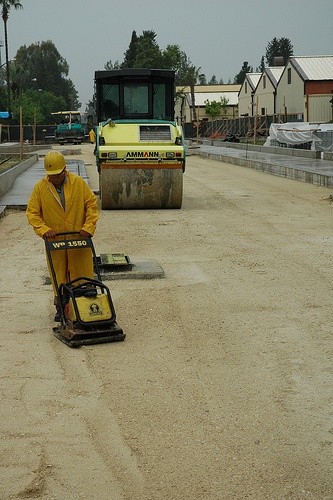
xmin=44 ymin=151 xmax=66 ymax=175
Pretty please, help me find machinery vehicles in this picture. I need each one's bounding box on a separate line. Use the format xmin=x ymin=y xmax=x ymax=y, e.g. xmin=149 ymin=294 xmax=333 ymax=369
xmin=88 ymin=69 xmax=189 ymax=211
xmin=50 ymin=111 xmax=85 ymax=145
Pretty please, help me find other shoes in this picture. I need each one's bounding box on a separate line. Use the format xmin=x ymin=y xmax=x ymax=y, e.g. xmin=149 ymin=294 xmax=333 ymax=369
xmin=54 ymin=305 xmax=61 ymax=322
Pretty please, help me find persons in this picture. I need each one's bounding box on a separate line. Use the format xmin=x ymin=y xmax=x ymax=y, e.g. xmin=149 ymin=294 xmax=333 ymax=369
xmin=89 ymin=129 xmax=96 ymax=144
xmin=26 ymin=150 xmax=100 ymax=321
